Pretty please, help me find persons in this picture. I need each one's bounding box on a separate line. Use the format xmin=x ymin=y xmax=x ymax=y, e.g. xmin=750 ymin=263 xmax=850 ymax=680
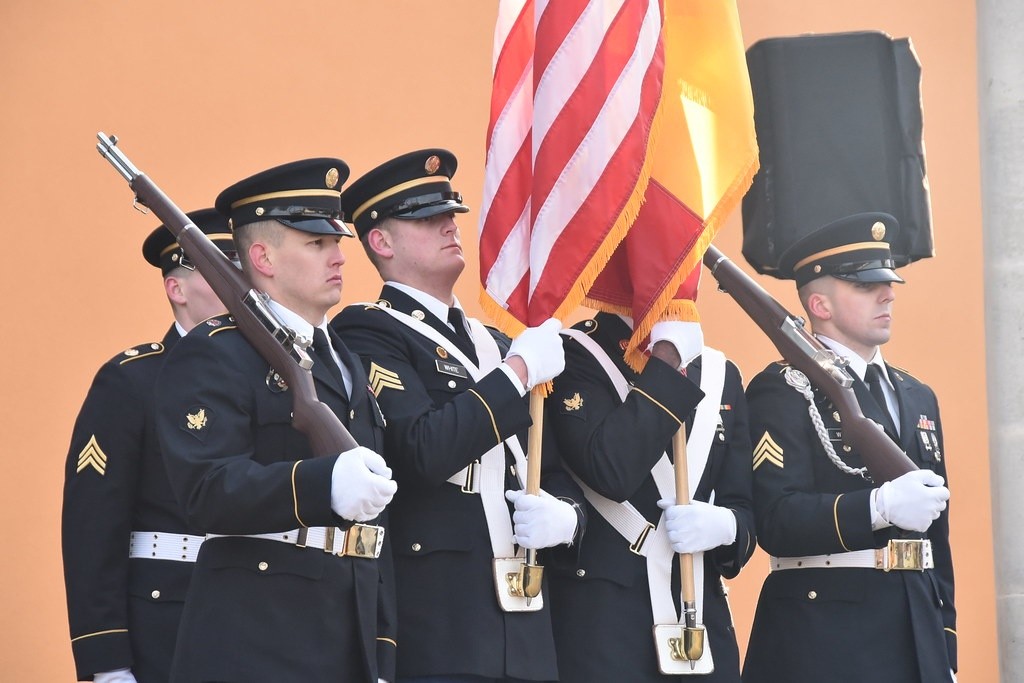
xmin=549 ymin=311 xmax=756 ymax=683
xmin=61 ymin=207 xmax=245 ymax=682
xmin=332 ymin=148 xmax=587 ymax=682
xmin=156 ymin=158 xmax=396 ymax=682
xmin=741 ymin=211 xmax=957 ymax=683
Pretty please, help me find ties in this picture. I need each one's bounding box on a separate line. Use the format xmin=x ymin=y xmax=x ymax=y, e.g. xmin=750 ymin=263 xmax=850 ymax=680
xmin=448 ymin=308 xmax=475 ymax=350
xmin=312 ymin=327 xmax=349 ymax=402
xmin=864 ymin=364 xmax=900 ymax=441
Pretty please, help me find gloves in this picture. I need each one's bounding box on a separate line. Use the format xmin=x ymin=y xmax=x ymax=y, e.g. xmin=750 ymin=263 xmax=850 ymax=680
xmin=92 ymin=668 xmax=137 ymax=683
xmin=647 ymin=314 xmax=704 ymax=372
xmin=656 ymin=497 xmax=737 ymax=554
xmin=874 ymin=470 xmax=950 ymax=533
xmin=504 ymin=487 xmax=577 ymax=549
xmin=503 ymin=318 xmax=566 ymax=392
xmin=329 ymin=447 xmax=398 ymax=523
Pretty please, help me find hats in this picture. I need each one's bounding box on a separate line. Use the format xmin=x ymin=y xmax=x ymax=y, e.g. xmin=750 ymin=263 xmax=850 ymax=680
xmin=215 ymin=158 xmax=354 ymax=238
xmin=141 ymin=207 xmax=243 ymax=276
xmin=340 ymin=148 xmax=470 ymax=240
xmin=779 ymin=212 xmax=905 ymax=289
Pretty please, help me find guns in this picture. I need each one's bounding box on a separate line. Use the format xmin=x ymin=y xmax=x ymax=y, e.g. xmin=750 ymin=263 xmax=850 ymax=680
xmin=703 ymin=243 xmax=923 ymax=485
xmin=96 ymin=130 xmax=360 ymax=457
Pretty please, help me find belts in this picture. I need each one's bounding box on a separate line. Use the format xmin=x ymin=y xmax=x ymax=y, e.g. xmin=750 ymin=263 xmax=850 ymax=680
xmin=769 ymin=537 xmax=934 ymax=572
xmin=128 ymin=532 xmax=206 ymax=562
xmin=205 ymin=522 xmax=384 ymax=559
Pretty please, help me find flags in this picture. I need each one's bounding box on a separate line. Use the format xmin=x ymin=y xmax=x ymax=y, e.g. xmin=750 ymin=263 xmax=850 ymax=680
xmin=478 ymin=0 xmax=761 ymax=397
